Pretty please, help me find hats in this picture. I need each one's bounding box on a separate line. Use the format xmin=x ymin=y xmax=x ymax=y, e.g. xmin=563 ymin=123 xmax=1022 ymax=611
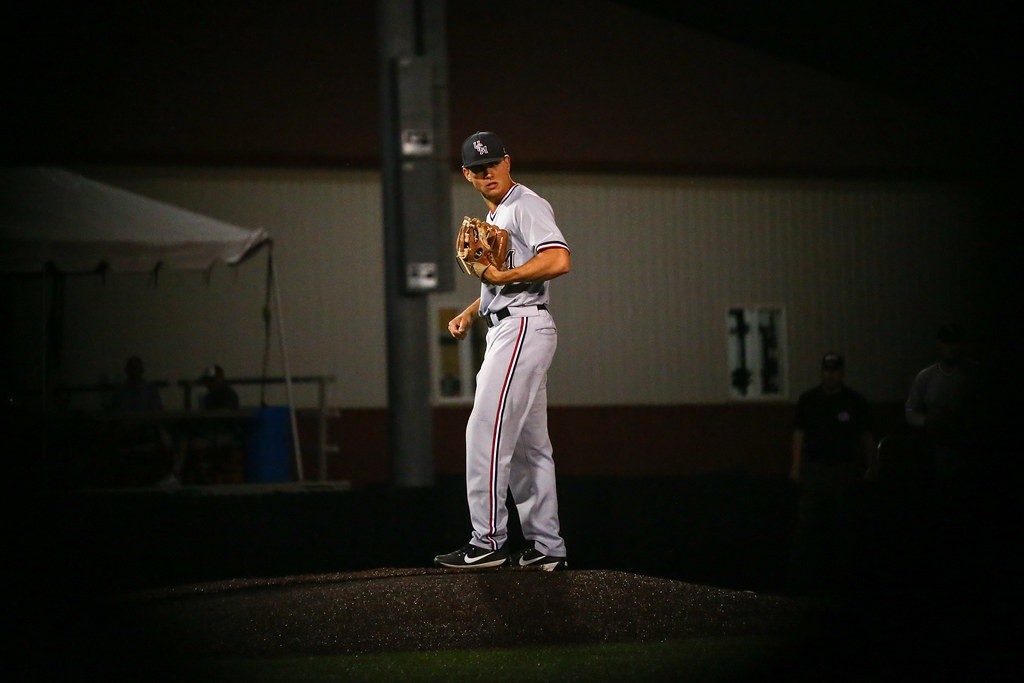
xmin=461 ymin=131 xmax=506 ymax=168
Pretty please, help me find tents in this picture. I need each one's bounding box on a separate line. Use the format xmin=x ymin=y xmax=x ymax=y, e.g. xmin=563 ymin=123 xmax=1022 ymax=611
xmin=0 ymin=164 xmax=274 ymax=410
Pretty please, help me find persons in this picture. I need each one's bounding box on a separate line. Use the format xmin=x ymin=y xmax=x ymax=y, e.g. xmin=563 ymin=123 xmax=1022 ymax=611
xmin=435 ymin=130 xmax=571 ymax=572
xmin=905 ymin=325 xmax=975 ymax=479
xmin=788 ymin=350 xmax=877 ymax=598
xmin=100 ymin=356 xmax=242 ymax=411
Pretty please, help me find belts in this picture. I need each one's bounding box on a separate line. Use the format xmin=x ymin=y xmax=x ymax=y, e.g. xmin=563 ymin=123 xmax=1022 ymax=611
xmin=486 ymin=304 xmax=548 ymax=327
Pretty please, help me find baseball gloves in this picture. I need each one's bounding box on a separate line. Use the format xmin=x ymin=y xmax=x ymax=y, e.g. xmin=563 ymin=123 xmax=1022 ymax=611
xmin=456 ymin=216 xmax=508 ymax=279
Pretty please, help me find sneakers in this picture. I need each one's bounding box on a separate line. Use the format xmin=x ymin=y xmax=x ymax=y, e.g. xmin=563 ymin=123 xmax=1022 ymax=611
xmin=432 ymin=542 xmax=509 ymax=570
xmin=503 ymin=548 xmax=568 ymax=573
